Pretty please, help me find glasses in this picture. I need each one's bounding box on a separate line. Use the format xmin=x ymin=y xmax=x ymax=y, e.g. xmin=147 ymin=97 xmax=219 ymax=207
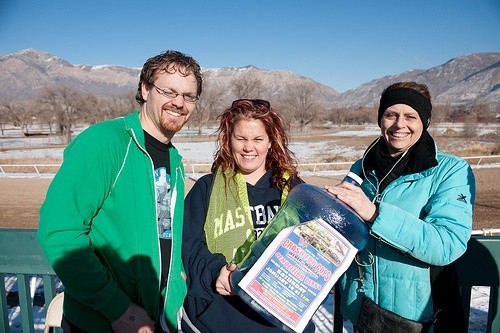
xmin=232 ymin=99 xmax=271 ymax=110
xmin=149 ymin=83 xmax=199 ymax=102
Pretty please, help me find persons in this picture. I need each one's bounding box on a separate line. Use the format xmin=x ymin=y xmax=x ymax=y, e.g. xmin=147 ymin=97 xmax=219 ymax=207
xmin=324 ymin=80 xmax=477 ymax=333
xmin=179 ymin=98 xmax=316 ymax=333
xmin=37 ymin=49 xmax=204 ymax=333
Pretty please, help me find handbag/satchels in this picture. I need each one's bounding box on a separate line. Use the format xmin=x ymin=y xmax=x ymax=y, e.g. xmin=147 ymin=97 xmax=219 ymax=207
xmin=434 ymin=238 xmax=499 ymax=333
xmin=353 ymin=296 xmax=432 ymax=333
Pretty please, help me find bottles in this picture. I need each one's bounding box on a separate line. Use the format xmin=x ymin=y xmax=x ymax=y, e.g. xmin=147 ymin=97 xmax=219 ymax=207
xmin=226 ymin=171 xmax=374 ymax=333
